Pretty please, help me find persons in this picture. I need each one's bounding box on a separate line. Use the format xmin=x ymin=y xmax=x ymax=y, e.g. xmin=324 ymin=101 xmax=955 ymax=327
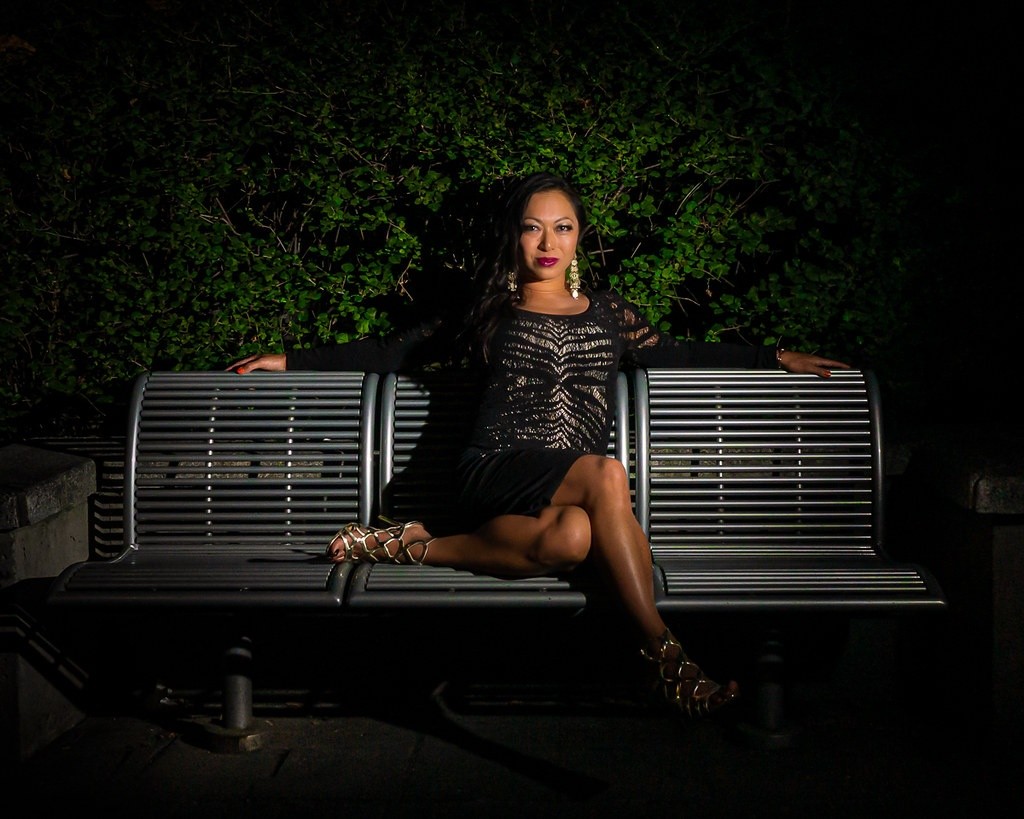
xmin=224 ymin=172 xmax=852 ymax=718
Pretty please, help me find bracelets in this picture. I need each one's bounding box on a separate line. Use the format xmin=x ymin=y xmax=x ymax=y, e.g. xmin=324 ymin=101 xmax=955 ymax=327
xmin=777 ymin=347 xmax=784 ymax=369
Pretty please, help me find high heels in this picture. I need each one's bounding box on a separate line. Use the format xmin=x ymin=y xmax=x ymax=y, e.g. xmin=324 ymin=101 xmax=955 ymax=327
xmin=323 ymin=515 xmax=441 ymax=568
xmin=640 ymin=625 xmax=738 ymax=726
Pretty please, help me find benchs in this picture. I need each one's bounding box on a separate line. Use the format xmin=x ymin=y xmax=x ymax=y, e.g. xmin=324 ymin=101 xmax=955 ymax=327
xmin=40 ymin=366 xmax=949 ymax=734
